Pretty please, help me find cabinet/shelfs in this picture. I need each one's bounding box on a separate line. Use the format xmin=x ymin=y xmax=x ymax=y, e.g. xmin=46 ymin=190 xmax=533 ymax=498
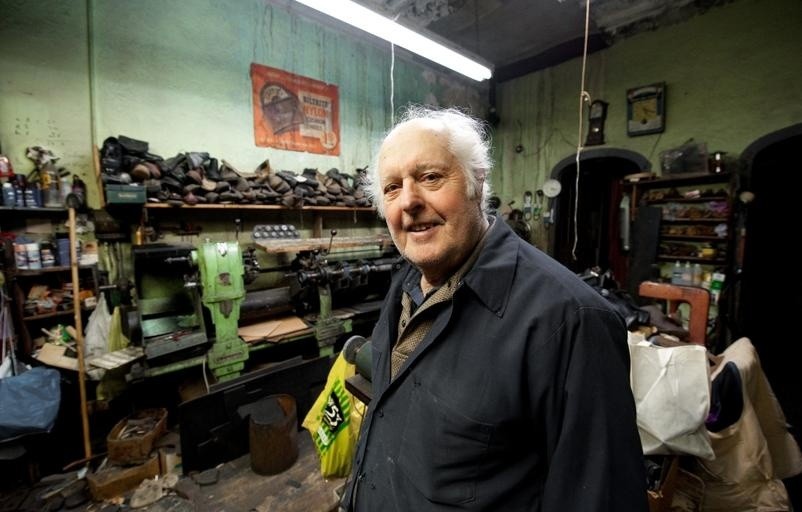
xmin=0 ymin=202 xmax=403 ymax=416
xmin=629 ymin=172 xmax=741 ymax=352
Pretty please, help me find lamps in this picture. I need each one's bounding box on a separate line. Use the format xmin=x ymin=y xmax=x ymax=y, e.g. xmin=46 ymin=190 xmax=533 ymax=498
xmin=291 ymin=0 xmax=496 ymax=84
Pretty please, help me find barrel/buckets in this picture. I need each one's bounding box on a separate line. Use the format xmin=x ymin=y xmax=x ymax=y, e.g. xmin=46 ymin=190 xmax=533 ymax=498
xmin=673 ymin=261 xmax=712 ymax=292
xmin=249 ymin=394 xmax=297 ymax=476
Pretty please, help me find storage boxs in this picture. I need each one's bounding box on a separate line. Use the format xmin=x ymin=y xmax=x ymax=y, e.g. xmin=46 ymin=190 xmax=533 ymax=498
xmin=86 ymin=408 xmax=183 ymax=502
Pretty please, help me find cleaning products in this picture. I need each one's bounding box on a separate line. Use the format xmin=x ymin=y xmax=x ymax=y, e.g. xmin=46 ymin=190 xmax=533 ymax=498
xmin=12 ymin=232 xmax=73 ymax=269
xmin=58 ymin=174 xmax=88 ymax=207
xmin=3 ymin=173 xmax=41 ymax=209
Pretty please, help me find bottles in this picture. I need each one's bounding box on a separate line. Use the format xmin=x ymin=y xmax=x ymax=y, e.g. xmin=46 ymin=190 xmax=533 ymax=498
xmin=669 ymin=261 xmax=702 ymax=289
xmin=710 ymin=151 xmax=729 ymax=173
xmin=14 ymin=232 xmax=69 ymax=269
xmin=2 ymin=160 xmax=85 ymax=211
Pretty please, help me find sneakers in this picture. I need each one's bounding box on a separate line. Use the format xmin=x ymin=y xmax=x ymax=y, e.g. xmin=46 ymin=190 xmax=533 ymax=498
xmin=674 ymin=206 xmax=711 ymax=218
xmin=650 ymin=188 xmax=727 ymax=202
xmin=221 ymin=160 xmax=372 ymax=207
xmin=661 ymin=227 xmax=717 ymax=236
xmin=102 ymin=136 xmax=244 ymax=202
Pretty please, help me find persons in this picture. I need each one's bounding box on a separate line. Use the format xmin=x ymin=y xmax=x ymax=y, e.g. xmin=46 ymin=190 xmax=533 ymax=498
xmin=341 ymin=111 xmax=651 ymax=512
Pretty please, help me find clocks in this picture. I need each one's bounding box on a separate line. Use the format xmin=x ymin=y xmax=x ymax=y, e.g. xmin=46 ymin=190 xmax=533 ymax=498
xmin=626 ymin=81 xmax=667 ymax=137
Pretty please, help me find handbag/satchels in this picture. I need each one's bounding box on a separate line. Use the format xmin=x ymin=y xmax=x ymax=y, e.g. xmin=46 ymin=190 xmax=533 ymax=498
xmin=626 ymin=328 xmax=716 ymax=462
